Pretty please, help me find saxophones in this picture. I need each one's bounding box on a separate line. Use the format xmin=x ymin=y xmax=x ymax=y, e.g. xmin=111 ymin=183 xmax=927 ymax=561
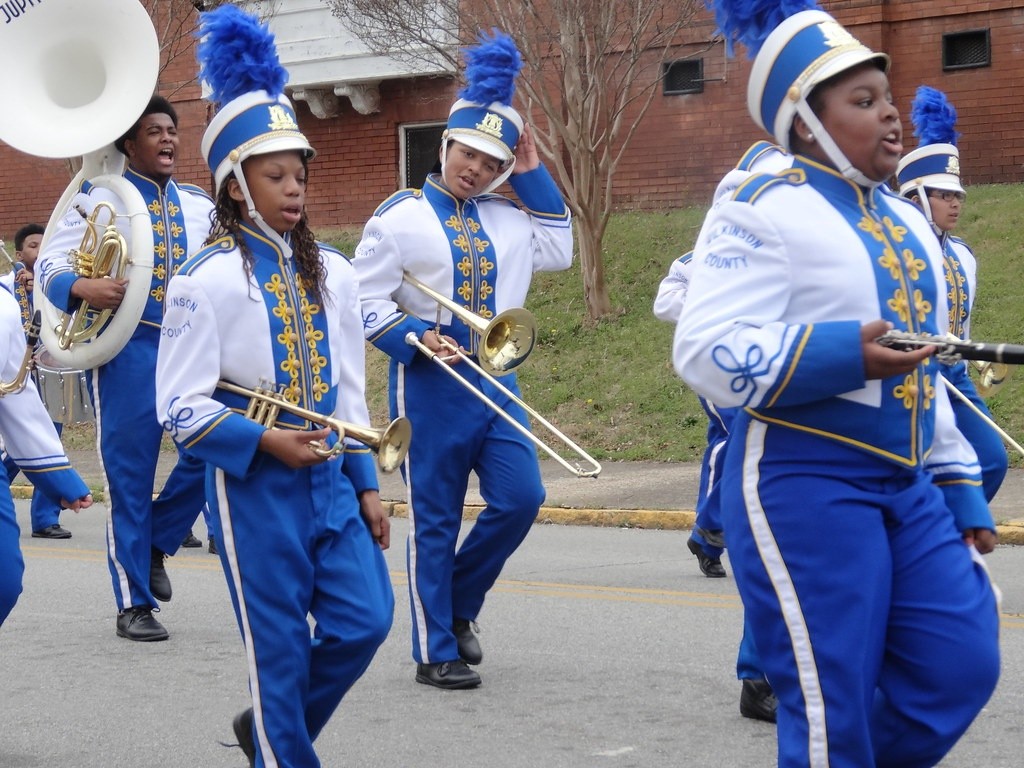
xmin=1 ymin=309 xmax=42 ymax=398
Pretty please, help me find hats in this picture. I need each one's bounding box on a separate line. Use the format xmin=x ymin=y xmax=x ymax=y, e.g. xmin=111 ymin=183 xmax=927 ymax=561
xmin=704 ymin=0 xmax=891 ymax=155
xmin=196 ymin=4 xmax=318 ymax=198
xmin=895 ymin=84 xmax=966 ymax=195
xmin=440 ymin=26 xmax=524 ymax=164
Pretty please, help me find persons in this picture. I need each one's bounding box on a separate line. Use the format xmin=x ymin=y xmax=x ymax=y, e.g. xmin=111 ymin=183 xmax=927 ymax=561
xmin=654 ymin=0 xmax=1009 ymax=768
xmin=0 ymin=95 xmax=223 ymax=641
xmin=156 ymin=4 xmax=399 ymax=768
xmin=349 ymin=26 xmax=572 ymax=689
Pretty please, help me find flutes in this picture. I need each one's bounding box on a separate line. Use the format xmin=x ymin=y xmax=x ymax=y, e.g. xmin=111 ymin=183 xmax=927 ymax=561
xmin=876 ymin=326 xmax=1024 ymax=368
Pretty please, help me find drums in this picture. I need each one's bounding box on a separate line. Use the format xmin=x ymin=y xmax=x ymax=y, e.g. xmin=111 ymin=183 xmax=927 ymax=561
xmin=33 ymin=343 xmax=95 ymax=426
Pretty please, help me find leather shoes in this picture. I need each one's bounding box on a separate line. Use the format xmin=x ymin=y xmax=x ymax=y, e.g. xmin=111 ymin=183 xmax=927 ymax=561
xmin=149 ymin=546 xmax=171 ymax=602
xmin=415 ymin=660 xmax=481 ymax=689
xmin=232 ymin=706 xmax=257 ymax=768
xmin=32 ymin=524 xmax=72 ymax=538
xmin=740 ymin=679 xmax=778 ymax=725
xmin=116 ymin=605 xmax=169 ymax=640
xmin=208 ymin=536 xmax=218 ymax=555
xmin=687 ymin=537 xmax=726 ymax=577
xmin=452 ymin=618 xmax=482 ymax=664
xmin=181 ymin=529 xmax=202 ymax=547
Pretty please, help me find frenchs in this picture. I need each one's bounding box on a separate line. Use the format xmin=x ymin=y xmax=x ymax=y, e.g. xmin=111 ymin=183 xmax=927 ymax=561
xmin=0 ymin=0 xmax=164 ymax=376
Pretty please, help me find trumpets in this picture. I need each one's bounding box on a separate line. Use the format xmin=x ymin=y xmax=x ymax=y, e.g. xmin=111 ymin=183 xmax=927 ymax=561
xmin=216 ymin=375 xmax=414 ymax=477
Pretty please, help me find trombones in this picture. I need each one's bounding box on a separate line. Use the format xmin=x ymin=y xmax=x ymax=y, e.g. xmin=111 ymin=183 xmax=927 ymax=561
xmin=935 ymin=331 xmax=1024 ymax=456
xmin=393 ymin=272 xmax=604 ymax=481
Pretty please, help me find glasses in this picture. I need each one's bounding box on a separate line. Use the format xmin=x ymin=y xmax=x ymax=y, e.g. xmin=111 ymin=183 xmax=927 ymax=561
xmin=928 ymin=191 xmax=965 ymax=203
